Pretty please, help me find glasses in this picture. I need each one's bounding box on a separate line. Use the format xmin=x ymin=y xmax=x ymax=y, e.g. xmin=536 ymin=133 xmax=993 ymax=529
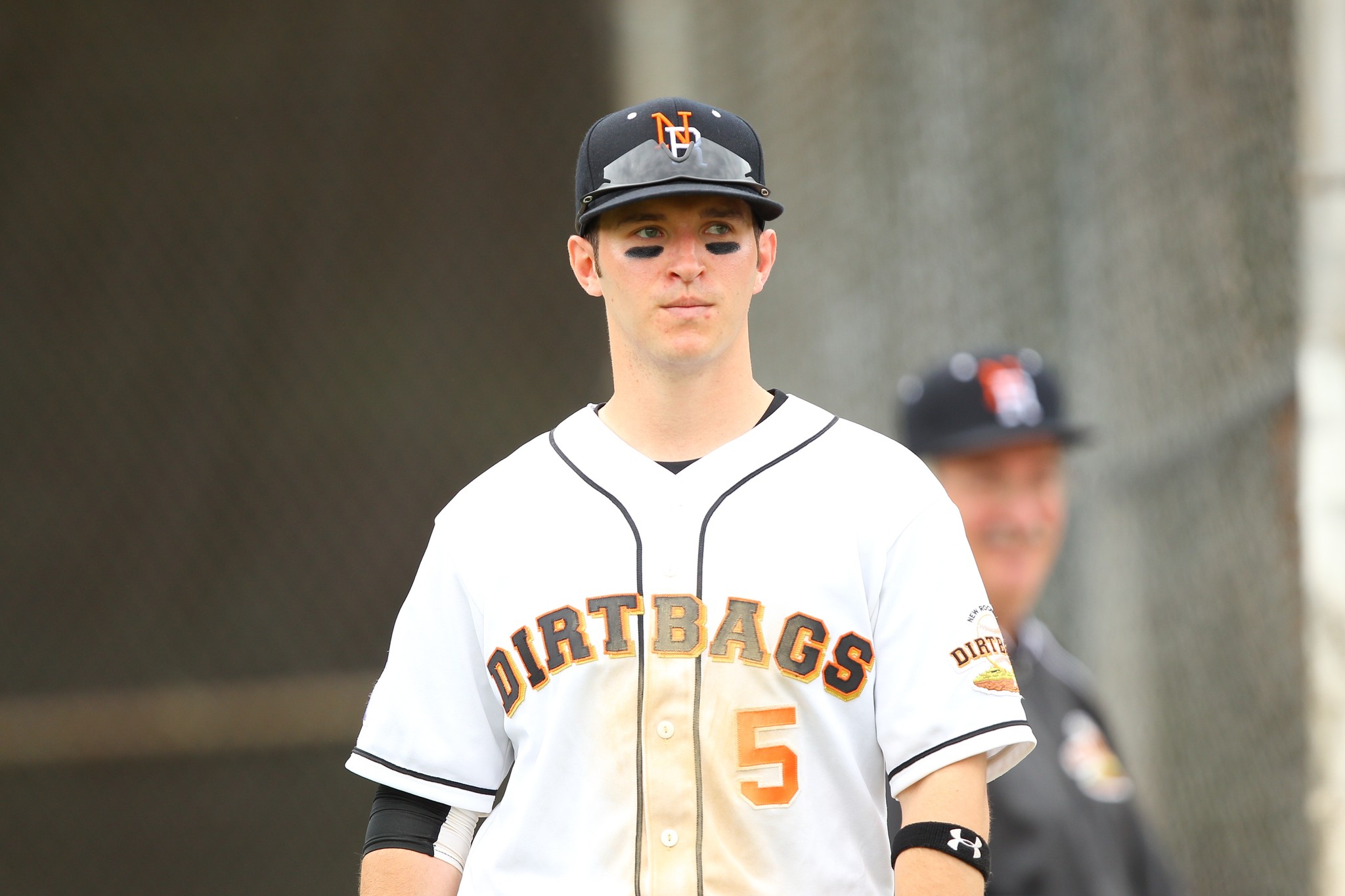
xmin=576 ymin=137 xmax=770 ymax=227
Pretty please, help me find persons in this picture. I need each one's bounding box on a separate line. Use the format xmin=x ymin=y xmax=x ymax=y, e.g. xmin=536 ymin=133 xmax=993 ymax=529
xmin=349 ymin=100 xmax=1033 ymax=895
xmin=894 ymin=353 xmax=1176 ymax=894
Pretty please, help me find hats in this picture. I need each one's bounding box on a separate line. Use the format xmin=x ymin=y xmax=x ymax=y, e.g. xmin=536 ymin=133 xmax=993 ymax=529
xmin=890 ymin=359 xmax=1087 ymax=462
xmin=577 ymin=97 xmax=784 ymax=236
